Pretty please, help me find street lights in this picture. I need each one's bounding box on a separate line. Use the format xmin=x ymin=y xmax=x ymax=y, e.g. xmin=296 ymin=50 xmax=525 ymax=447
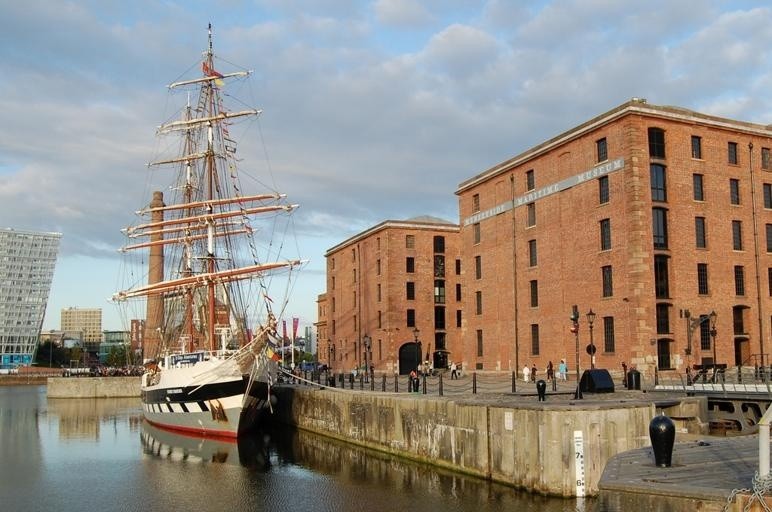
xmin=710 ymin=311 xmax=718 ymax=363
xmin=586 ymin=308 xmax=596 ymax=368
xmin=413 ymin=328 xmax=420 ymax=378
xmin=363 ymin=334 xmax=369 ymax=382
xmin=326 ymin=339 xmax=331 ymax=375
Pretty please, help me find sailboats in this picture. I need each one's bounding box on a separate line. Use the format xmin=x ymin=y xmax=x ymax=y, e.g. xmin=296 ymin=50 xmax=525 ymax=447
xmin=108 ymin=23 xmax=311 ymax=439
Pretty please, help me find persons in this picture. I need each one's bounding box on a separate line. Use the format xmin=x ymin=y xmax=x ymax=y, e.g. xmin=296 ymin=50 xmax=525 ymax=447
xmin=370 ymin=363 xmax=374 ymax=375
xmin=410 ymin=368 xmax=416 ymax=388
xmin=559 ymin=360 xmax=567 ymax=381
xmin=531 ymin=363 xmax=537 ymax=383
xmin=451 ymin=361 xmax=457 ymax=380
xmin=102 ymin=368 xmax=144 ymax=376
xmin=418 ymin=361 xmax=422 ymax=377
xmin=547 ymin=361 xmax=553 ymax=383
xmin=523 ymin=364 xmax=529 ymax=383
xmin=429 ymin=362 xmax=433 ymax=378
xmin=290 ymin=367 xmax=299 ymax=384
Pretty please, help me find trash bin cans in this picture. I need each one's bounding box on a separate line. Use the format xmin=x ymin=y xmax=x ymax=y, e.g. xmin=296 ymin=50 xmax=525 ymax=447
xmin=628 ymin=371 xmax=640 ymax=390
xmin=329 ymin=376 xmax=335 ymax=387
